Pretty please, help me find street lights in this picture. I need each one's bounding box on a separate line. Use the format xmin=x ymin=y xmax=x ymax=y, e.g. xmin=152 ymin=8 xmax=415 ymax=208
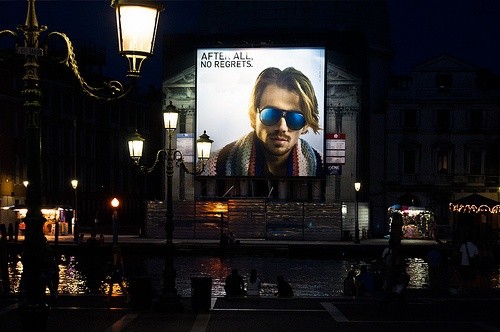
xmin=354 ymin=179 xmax=362 ymax=243
xmin=0 ymin=0 xmax=166 ymax=332
xmin=109 ymin=196 xmax=120 ymax=245
xmin=127 ymin=100 xmax=214 ymax=313
xmin=70 ymin=178 xmax=81 ymax=244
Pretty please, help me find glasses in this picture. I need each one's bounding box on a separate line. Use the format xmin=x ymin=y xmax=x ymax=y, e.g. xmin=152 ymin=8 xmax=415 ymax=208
xmin=257 ymin=105 xmax=308 ymax=130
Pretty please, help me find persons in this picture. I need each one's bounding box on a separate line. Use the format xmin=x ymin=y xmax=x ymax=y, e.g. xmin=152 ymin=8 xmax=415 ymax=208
xmin=276 ymin=275 xmax=295 ymax=297
xmin=460 ymin=232 xmax=500 ymax=288
xmin=343 ymin=263 xmax=386 ymax=297
xmin=202 ymin=67 xmax=324 ymax=177
xmin=106 ymin=244 xmax=130 ymax=305
xmin=246 ymin=269 xmax=261 ymax=296
xmin=76 ymin=232 xmax=105 ymax=246
xmin=220 ymin=228 xmax=237 ymax=248
xmin=222 ymin=268 xmax=247 ymax=297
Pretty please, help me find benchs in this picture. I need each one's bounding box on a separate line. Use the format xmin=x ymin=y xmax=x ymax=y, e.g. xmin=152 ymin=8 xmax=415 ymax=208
xmin=266 ymin=221 xmax=307 ymax=240
xmin=174 ymin=217 xmax=224 ymax=239
